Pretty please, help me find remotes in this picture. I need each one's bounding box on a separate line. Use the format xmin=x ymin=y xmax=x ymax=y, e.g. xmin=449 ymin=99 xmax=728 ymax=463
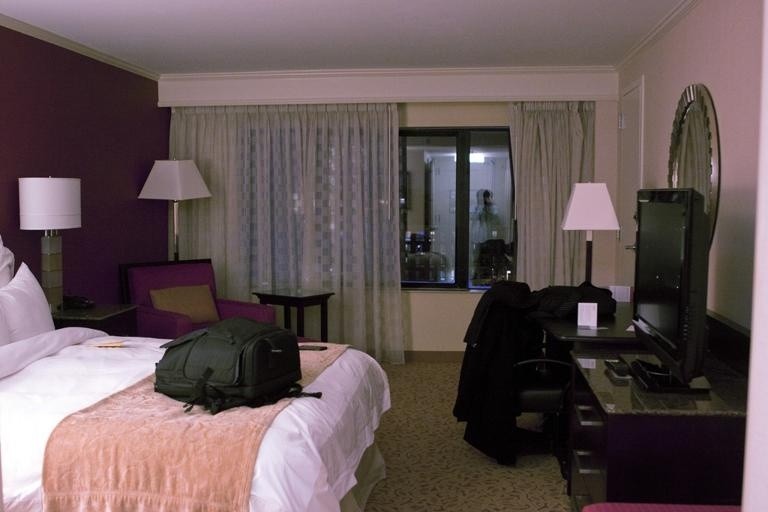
xmin=604 ymin=359 xmax=634 ymax=381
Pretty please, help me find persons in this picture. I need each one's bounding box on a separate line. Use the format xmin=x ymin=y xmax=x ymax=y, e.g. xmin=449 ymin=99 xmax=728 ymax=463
xmin=471 ymin=189 xmax=505 ymax=241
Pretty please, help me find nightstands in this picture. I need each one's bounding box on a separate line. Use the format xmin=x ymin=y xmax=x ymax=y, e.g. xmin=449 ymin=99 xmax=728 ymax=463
xmin=52 ymin=304 xmax=141 ymax=337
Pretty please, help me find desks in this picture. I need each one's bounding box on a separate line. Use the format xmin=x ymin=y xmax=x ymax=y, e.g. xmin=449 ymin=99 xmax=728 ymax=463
xmin=543 ymin=299 xmax=634 ymax=364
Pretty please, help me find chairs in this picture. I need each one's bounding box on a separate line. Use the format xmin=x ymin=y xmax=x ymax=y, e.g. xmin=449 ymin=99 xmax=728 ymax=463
xmin=116 ymin=257 xmax=276 ymax=339
xmin=452 ymin=282 xmax=592 ymax=455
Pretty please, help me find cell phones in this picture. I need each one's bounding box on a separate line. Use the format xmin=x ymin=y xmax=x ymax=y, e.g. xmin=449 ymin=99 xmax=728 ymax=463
xmin=299 ymin=345 xmax=328 ymax=351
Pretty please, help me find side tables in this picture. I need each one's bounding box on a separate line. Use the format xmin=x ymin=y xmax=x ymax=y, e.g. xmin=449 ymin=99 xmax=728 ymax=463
xmin=251 ymin=286 xmax=335 ymax=350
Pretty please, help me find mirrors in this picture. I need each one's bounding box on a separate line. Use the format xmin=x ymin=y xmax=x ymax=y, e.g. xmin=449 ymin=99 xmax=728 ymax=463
xmin=666 ymin=83 xmax=719 ymax=256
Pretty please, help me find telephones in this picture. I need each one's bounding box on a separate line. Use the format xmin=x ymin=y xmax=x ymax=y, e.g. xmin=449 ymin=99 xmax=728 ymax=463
xmin=63 ymin=295 xmax=94 ymax=311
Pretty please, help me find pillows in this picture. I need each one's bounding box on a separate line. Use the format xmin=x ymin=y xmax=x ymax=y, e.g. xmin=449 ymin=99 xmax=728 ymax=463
xmin=149 ymin=283 xmax=220 ymax=325
xmin=0 ymin=261 xmax=55 ymax=346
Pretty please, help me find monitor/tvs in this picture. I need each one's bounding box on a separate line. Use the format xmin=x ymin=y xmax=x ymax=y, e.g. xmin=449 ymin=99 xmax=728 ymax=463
xmin=617 ymin=187 xmax=712 ymax=392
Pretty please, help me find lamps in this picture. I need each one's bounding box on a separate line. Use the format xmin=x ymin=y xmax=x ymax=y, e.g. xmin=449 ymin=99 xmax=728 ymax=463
xmin=137 ymin=152 xmax=212 ymax=260
xmin=561 ymin=179 xmax=620 ymax=282
xmin=18 ymin=175 xmax=82 ymax=314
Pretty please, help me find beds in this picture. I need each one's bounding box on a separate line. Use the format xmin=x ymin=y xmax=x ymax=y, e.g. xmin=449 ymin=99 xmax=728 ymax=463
xmin=1 ymin=235 xmax=392 ymax=511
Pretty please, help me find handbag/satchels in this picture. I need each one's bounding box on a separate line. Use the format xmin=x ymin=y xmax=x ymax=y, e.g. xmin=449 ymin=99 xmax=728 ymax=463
xmin=155 ymin=317 xmax=302 ymax=415
xmin=527 ymin=281 xmax=616 ymax=321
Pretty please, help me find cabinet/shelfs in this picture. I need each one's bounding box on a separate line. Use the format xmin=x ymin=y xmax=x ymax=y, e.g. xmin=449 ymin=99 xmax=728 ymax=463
xmin=566 ymin=346 xmax=749 ymax=511
